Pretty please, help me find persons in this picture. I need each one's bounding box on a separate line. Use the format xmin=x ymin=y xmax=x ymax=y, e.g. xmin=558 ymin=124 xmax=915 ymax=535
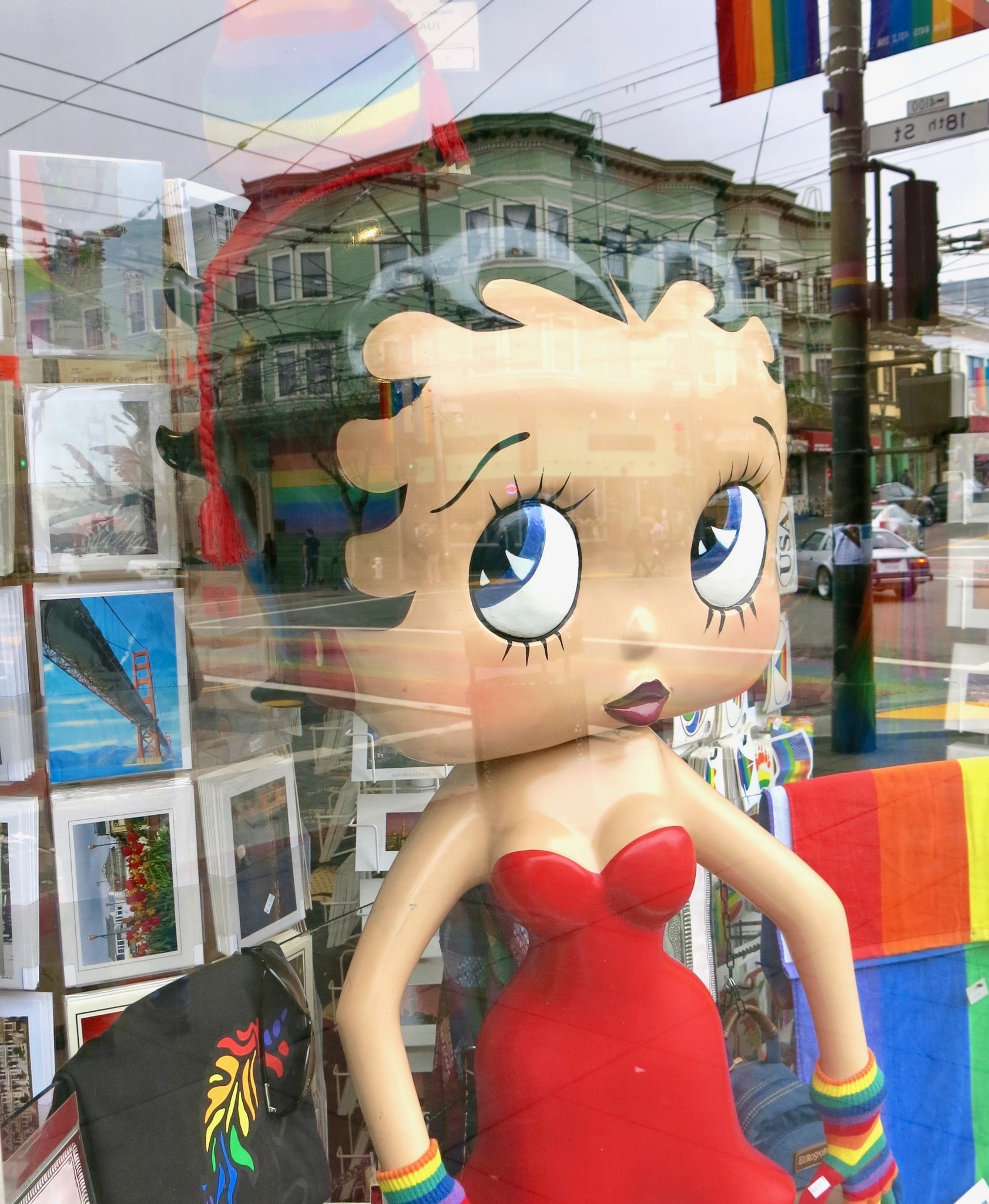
xmin=171 ymin=2 xmax=900 ymax=1204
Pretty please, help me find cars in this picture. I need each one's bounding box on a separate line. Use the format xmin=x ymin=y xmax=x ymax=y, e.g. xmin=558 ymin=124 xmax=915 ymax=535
xmin=824 ymin=503 xmax=926 ymax=551
xmin=929 ymin=483 xmax=986 ymax=522
xmin=796 ymin=527 xmax=934 ymax=600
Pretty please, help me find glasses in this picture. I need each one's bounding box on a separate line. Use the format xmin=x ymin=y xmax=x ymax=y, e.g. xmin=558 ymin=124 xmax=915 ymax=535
xmin=249 ymin=944 xmax=316 ymax=1117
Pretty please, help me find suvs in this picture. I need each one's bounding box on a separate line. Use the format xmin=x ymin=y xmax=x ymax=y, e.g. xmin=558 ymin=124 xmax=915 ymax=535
xmin=871 ymin=481 xmax=936 ymax=528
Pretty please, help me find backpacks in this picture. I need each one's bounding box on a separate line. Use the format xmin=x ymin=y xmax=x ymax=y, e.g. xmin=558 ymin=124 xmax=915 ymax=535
xmin=723 ymin=1004 xmax=829 ymax=1188
